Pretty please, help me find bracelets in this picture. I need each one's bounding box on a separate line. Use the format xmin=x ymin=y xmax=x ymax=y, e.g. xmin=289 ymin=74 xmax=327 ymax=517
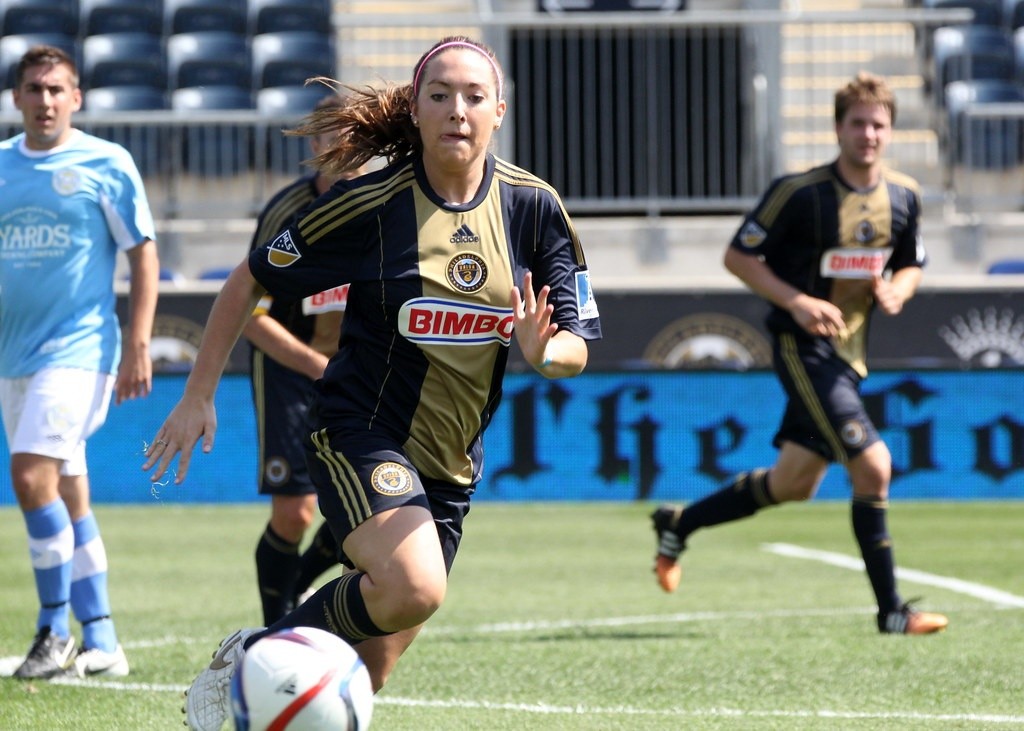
xmin=531 ymin=353 xmax=552 ymax=369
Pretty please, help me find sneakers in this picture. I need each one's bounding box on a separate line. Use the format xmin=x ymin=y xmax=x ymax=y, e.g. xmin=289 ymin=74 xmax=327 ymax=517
xmin=877 ymin=595 xmax=948 ymax=635
xmin=11 ymin=625 xmax=80 ymax=679
xmin=181 ymin=627 xmax=270 ymax=731
xmin=650 ymin=503 xmax=687 ymax=592
xmin=48 ymin=643 xmax=130 ymax=683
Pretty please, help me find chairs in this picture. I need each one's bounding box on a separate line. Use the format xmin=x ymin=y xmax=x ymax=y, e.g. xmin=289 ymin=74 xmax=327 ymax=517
xmin=923 ymin=0 xmax=1024 ymax=213
xmin=0 ymin=1 xmax=341 ymax=233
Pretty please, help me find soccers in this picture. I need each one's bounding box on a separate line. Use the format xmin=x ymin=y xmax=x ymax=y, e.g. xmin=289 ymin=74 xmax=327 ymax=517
xmin=229 ymin=625 xmax=373 ymax=731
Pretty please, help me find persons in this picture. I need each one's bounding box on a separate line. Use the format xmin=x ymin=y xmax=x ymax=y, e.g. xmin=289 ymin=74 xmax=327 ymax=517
xmin=648 ymin=71 xmax=948 ymax=634
xmin=1 ymin=48 xmax=161 ymax=685
xmin=141 ymin=36 xmax=604 ymax=731
xmin=243 ymin=98 xmax=369 ymax=632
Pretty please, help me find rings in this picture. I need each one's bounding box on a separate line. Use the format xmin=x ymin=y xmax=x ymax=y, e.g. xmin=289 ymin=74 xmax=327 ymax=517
xmin=157 ymin=440 xmax=168 ymax=446
